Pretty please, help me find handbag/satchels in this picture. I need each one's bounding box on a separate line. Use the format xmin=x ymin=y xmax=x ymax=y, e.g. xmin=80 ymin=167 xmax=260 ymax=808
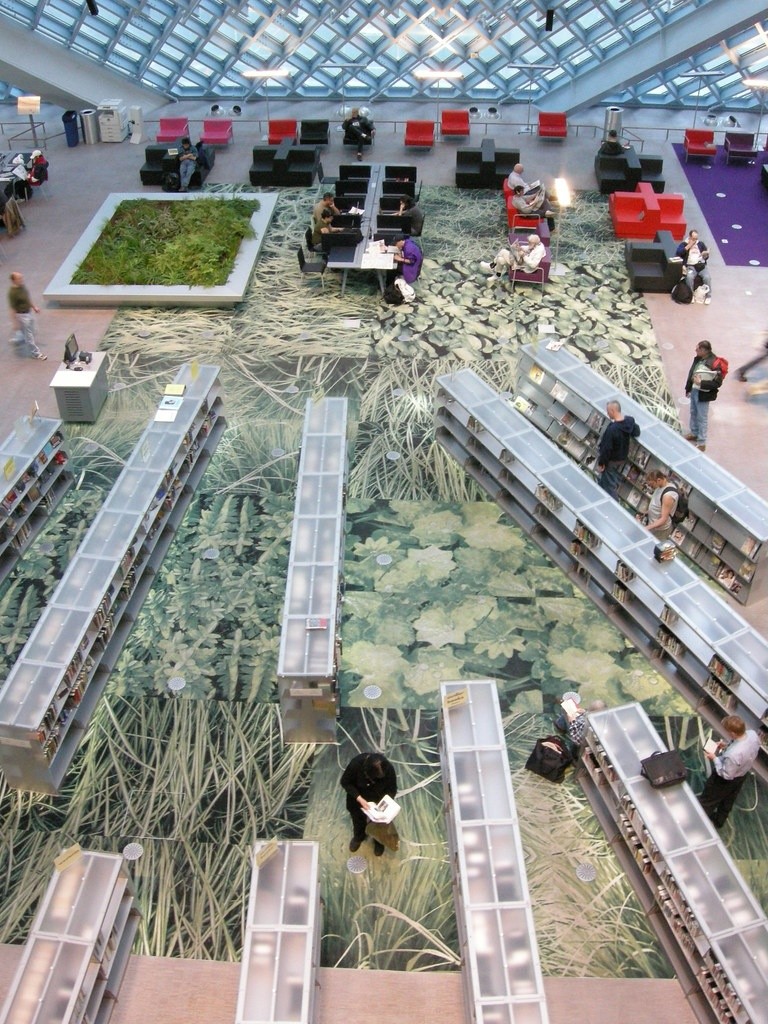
xmin=366 ymin=821 xmax=400 ymax=851
xmin=671 ymin=283 xmax=693 ymax=304
xmin=524 ymin=735 xmax=571 ymax=784
xmin=641 ymin=749 xmax=688 ymax=790
xmin=694 ymin=283 xmax=709 ymax=304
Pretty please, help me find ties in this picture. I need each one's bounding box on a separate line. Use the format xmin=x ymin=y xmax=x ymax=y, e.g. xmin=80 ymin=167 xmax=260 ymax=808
xmin=724 ymin=739 xmax=734 ymax=752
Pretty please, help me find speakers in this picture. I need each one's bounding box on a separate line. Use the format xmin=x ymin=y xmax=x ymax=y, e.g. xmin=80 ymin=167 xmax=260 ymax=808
xmin=86 ymin=0 xmax=99 ymax=15
xmin=546 ymin=10 xmax=553 ymax=31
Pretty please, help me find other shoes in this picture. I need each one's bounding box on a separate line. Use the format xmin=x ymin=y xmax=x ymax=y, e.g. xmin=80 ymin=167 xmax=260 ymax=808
xmin=364 ymin=135 xmax=371 ymax=140
xmin=374 ymin=839 xmax=384 ymax=856
xmin=32 ymin=353 xmax=47 ymax=360
xmin=487 ymin=274 xmax=503 ymax=281
xmin=349 ymin=838 xmax=361 ymax=852
xmin=683 ymin=433 xmax=697 ymax=441
xmin=545 ymin=212 xmax=557 ymax=217
xmin=480 ymin=262 xmax=495 ymax=274
xmin=180 ymin=187 xmax=187 ymax=192
xmin=705 ymin=298 xmax=711 ymax=305
xmin=695 ymin=444 xmax=705 ymax=451
xmin=358 ymin=153 xmax=362 ymax=162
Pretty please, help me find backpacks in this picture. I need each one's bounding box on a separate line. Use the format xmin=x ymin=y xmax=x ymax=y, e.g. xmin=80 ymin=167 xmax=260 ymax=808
xmin=384 ymin=283 xmax=404 ymax=306
xmin=162 ymin=171 xmax=179 ymax=192
xmin=713 ymin=358 xmax=729 ymax=380
xmin=394 ymin=279 xmax=415 ymax=304
xmin=661 ymin=482 xmax=689 ymax=523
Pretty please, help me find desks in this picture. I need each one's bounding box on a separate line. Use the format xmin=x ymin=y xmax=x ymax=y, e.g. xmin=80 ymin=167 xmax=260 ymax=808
xmin=0 ymin=413 xmax=77 ymax=586
xmin=298 ymin=161 xmax=424 ymax=297
xmin=0 ymin=151 xmax=34 ymax=208
xmin=427 ymin=364 xmax=767 ymax=761
xmin=50 ymin=351 xmax=109 ymax=424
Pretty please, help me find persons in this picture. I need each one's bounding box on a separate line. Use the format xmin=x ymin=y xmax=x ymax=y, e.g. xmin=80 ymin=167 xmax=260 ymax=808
xmin=599 ymin=400 xmax=640 ymax=502
xmin=8 ymin=271 xmax=46 ymax=360
xmin=553 ymin=701 xmax=606 ymax=743
xmin=696 ymin=717 xmax=761 ymax=827
xmin=685 ymin=340 xmax=723 ymax=453
xmin=509 ymin=164 xmax=559 ymax=231
xmin=313 ymin=191 xmax=343 ymax=273
xmin=642 ymin=469 xmax=679 ymax=540
xmin=676 ymin=230 xmax=711 ymax=304
xmin=339 ymin=752 xmax=397 ymax=856
xmin=15 ymin=150 xmax=49 ymax=204
xmin=343 ymin=108 xmax=371 ymax=161
xmin=394 ymin=194 xmax=423 ymax=235
xmin=177 ymin=139 xmax=199 ymax=191
xmin=387 ymin=235 xmax=423 ymax=285
xmin=481 ymin=235 xmax=546 ymax=281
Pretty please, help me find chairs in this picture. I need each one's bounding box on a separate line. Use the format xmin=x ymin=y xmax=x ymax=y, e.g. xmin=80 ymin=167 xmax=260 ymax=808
xmin=407 ymin=179 xmax=423 ymax=208
xmin=305 ymin=216 xmax=321 ymax=257
xmin=304 ymin=225 xmax=329 ymax=261
xmin=401 ymin=275 xmax=424 ymax=297
xmin=403 ymin=216 xmax=426 ymax=252
xmin=318 ymin=162 xmax=339 ymax=193
xmin=298 ymin=246 xmax=326 ymax=293
xmin=0 ymin=194 xmax=26 ymax=236
xmin=503 ymin=177 xmax=556 ymax=301
xmin=19 ymin=162 xmax=49 ymax=203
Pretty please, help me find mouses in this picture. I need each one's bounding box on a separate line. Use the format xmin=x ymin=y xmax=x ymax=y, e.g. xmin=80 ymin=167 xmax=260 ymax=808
xmin=73 ymin=367 xmax=83 ymax=371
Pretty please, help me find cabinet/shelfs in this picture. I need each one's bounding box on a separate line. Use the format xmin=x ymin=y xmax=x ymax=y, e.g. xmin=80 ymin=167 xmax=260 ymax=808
xmin=511 ymin=341 xmax=768 ymax=610
xmin=275 ymin=392 xmax=347 ymax=748
xmin=573 ymin=702 xmax=768 ymax=1023
xmin=233 ymin=836 xmax=325 ymax=1023
xmin=440 ymin=677 xmax=550 ymax=1023
xmin=0 ymin=364 xmax=228 ymax=794
xmin=1 ymin=847 xmax=143 ymax=1023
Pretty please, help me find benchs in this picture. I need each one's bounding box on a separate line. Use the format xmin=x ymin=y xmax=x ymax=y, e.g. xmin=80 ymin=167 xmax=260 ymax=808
xmin=403 ymin=121 xmax=435 ymax=149
xmin=139 ymin=140 xmax=216 ymax=187
xmin=538 ymin=112 xmax=568 ymax=139
xmin=344 ymin=121 xmax=374 ymax=147
xmin=155 ymin=115 xmax=189 ymax=143
xmin=199 ymin=118 xmax=235 ymax=146
xmin=623 ymin=230 xmax=694 ymax=296
xmin=455 ymin=136 xmax=522 ymax=187
xmin=249 ymin=138 xmax=320 ymax=186
xmin=609 ymin=183 xmax=687 ymax=241
xmin=723 ymin=131 xmax=758 ymax=163
xmin=268 ymin=119 xmax=294 ymax=143
xmin=683 ymin=128 xmax=717 ymax=164
xmin=298 ymin=117 xmax=329 ymax=146
xmin=593 ymin=143 xmax=666 ymax=191
xmin=441 ymin=110 xmax=471 ymax=137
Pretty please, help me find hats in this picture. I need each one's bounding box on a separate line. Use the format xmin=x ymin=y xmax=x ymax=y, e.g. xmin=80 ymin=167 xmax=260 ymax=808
xmin=30 ymin=150 xmax=43 ymax=159
xmin=320 ymin=208 xmax=333 ymax=217
xmin=390 ymin=234 xmax=404 ymax=245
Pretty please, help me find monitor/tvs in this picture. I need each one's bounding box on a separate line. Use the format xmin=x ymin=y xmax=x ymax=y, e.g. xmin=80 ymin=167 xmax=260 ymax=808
xmin=63 ymin=334 xmax=79 ymax=363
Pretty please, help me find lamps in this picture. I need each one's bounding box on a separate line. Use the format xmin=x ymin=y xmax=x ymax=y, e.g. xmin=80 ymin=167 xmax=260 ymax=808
xmin=240 ymin=69 xmax=290 ymax=141
xmin=741 ymin=78 xmax=768 ymax=147
xmin=321 ymin=62 xmax=368 ymax=132
xmin=507 ymin=63 xmax=559 ymax=132
xmin=676 ymin=70 xmax=726 ymax=126
xmin=413 ymin=69 xmax=464 ymax=141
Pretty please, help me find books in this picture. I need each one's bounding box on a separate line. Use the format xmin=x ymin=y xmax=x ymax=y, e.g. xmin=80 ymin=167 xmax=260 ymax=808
xmin=168 ymin=149 xmax=178 ymax=155
xmin=1 ymin=339 xmax=767 ymax=1023
xmin=702 ymin=247 xmax=711 ymax=255
xmin=669 ymin=257 xmax=683 ymax=262
xmin=560 ymin=698 xmax=582 ymax=718
xmin=703 ymin=738 xmax=719 ymax=755
xmin=361 ymin=795 xmax=401 ymax=825
